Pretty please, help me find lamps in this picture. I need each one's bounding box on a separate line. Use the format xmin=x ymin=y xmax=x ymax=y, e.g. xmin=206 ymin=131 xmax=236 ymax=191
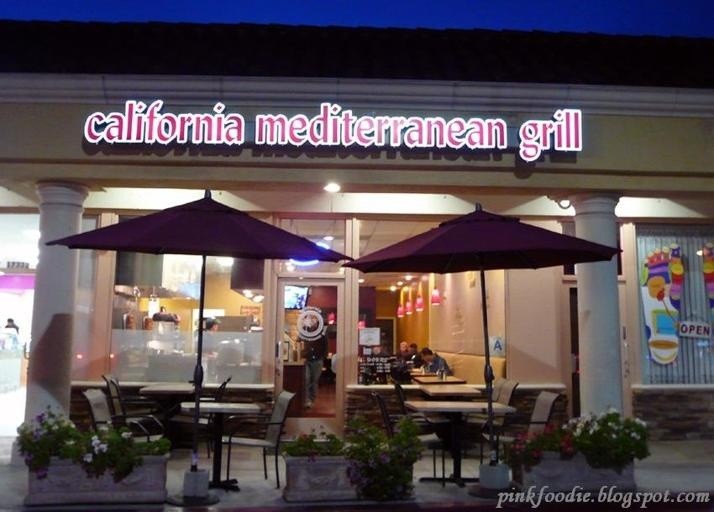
xmin=394 ymin=268 xmax=448 ymax=322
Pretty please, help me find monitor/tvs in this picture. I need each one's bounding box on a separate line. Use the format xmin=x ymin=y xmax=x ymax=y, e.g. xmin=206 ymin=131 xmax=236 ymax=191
xmin=284 ymin=285 xmax=309 ymax=310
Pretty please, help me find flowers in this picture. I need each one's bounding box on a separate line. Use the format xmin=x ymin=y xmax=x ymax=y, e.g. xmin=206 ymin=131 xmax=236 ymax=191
xmin=15 ymin=403 xmax=171 ymax=480
xmin=282 ymin=411 xmax=420 ymax=500
xmin=502 ymin=403 xmax=653 ymax=477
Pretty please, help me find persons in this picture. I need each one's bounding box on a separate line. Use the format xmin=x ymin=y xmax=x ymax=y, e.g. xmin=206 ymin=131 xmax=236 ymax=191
xmin=331 ymin=341 xmax=452 ymax=385
xmin=203 ymin=320 xmax=219 ymax=382
xmin=5 ymin=318 xmax=18 ymax=334
xmin=295 ymin=315 xmax=328 ymax=409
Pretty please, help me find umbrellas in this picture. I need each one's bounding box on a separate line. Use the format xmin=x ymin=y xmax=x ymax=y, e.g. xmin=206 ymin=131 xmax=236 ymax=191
xmin=46 ymin=190 xmax=355 ymax=471
xmin=341 ymin=203 xmax=623 ymax=466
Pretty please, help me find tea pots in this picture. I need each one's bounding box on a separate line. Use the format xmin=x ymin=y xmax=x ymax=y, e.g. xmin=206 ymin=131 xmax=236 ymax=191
xmin=419 ymin=364 xmax=430 ymax=375
xmin=436 ymin=369 xmax=447 ymax=380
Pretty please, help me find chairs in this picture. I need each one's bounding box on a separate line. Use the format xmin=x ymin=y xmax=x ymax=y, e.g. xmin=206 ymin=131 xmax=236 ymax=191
xmin=60 ymin=375 xmax=299 ymax=494
xmin=361 ymin=351 xmax=564 ymax=497
